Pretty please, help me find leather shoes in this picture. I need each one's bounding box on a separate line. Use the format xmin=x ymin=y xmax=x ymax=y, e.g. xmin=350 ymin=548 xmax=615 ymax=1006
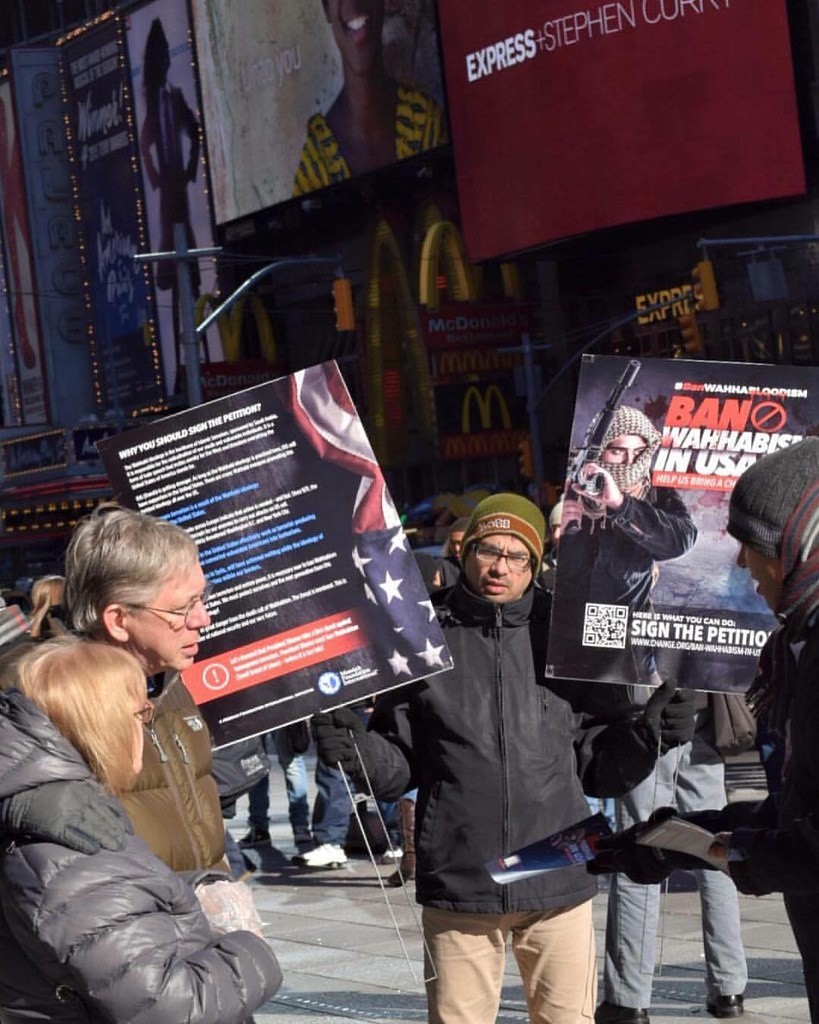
xmin=595 ymin=1000 xmax=648 ymax=1024
xmin=702 ymin=991 xmax=747 ymax=1018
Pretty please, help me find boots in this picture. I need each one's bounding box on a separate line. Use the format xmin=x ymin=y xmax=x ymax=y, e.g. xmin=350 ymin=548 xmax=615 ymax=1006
xmin=385 ymin=797 xmax=418 ymax=887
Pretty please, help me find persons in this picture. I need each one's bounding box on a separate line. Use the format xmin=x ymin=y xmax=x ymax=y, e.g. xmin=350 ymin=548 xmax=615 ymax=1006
xmin=235 ymin=724 xmax=311 ymax=850
xmin=0 ymin=633 xmax=282 ymax=1024
xmin=139 ymin=13 xmax=211 ymax=365
xmin=589 ymin=434 xmax=819 ymax=1024
xmin=67 ymin=503 xmax=226 ymax=871
xmin=412 ymin=502 xmax=433 ymax=546
xmin=434 ymin=517 xmax=470 ymax=588
xmin=292 ymin=700 xmax=403 ymax=866
xmin=596 ymin=688 xmax=750 ymax=1024
xmin=388 ymin=787 xmax=417 ymax=886
xmin=295 ymin=2 xmax=441 ymax=196
xmin=549 ymin=404 xmax=697 ymax=687
xmin=541 ymin=494 xmax=564 ymax=595
xmin=310 ymin=491 xmax=694 ymax=1024
xmin=28 ymin=575 xmax=67 ymax=640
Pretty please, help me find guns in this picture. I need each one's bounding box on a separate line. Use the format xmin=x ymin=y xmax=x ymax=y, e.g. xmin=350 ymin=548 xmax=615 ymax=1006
xmin=563 ymin=359 xmax=641 ymax=537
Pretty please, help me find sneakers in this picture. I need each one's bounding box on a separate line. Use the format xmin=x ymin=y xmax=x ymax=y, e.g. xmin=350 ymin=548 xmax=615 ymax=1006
xmin=293 ymin=823 xmax=310 ymax=843
xmin=237 ymin=828 xmax=270 ymax=850
xmin=289 ymin=840 xmax=350 ymax=869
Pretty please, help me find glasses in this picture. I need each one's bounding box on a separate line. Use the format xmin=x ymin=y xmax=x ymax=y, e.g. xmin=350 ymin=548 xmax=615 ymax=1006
xmin=110 ymin=579 xmax=216 ymax=625
xmin=128 ymin=696 xmax=155 ymax=726
xmin=460 ymin=538 xmax=533 ymax=574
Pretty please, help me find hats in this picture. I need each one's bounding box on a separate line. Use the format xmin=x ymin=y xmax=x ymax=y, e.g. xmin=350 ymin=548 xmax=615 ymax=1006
xmin=727 ymin=434 xmax=818 ymax=558
xmin=455 ymin=496 xmax=547 ymax=576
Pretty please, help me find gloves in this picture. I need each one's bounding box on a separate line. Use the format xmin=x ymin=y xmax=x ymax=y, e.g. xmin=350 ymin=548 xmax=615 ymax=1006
xmin=636 ymin=676 xmax=700 ymax=754
xmin=309 ymin=706 xmax=369 ymax=775
xmin=581 ymin=808 xmax=679 ymax=886
